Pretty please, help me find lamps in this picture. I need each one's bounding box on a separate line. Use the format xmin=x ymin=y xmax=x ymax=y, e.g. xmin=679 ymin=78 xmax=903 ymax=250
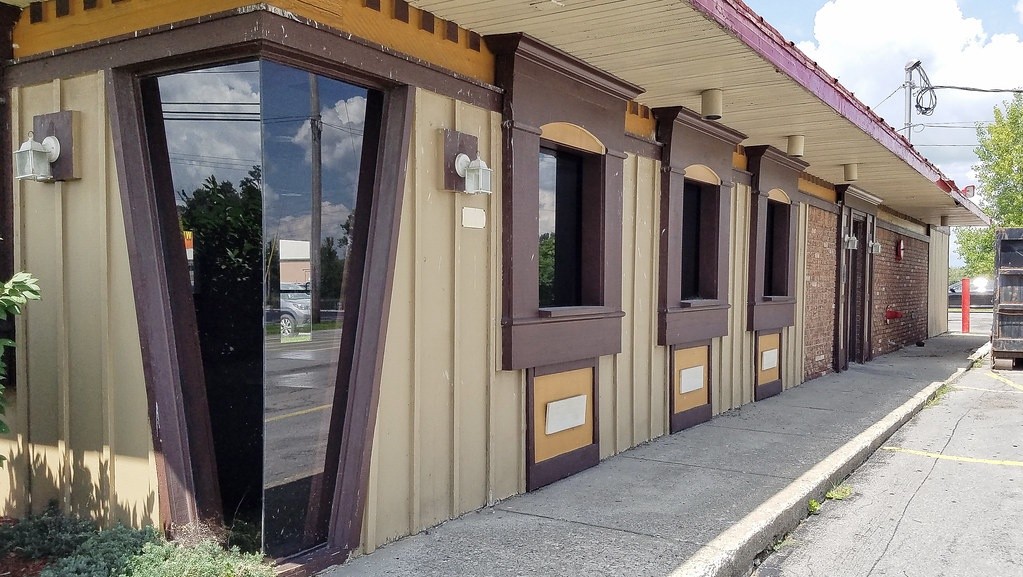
xmin=454 ymin=151 xmax=494 ymax=196
xmin=787 ymin=135 xmax=804 ymax=157
xmin=844 ymin=233 xmax=858 ymax=250
xmin=701 ymin=89 xmax=722 ymax=120
xmin=869 ymin=240 xmax=881 ymax=255
xmin=844 ymin=163 xmax=858 ymax=181
xmin=13 ymin=131 xmax=60 ymax=181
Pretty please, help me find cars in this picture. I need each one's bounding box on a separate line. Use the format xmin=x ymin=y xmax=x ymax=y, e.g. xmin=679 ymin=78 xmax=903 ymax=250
xmin=949 ymin=274 xmax=996 ymax=306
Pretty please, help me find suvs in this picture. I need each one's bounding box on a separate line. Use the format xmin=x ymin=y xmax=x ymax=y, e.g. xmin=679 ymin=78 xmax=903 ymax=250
xmin=263 ymin=281 xmax=313 ymax=336
